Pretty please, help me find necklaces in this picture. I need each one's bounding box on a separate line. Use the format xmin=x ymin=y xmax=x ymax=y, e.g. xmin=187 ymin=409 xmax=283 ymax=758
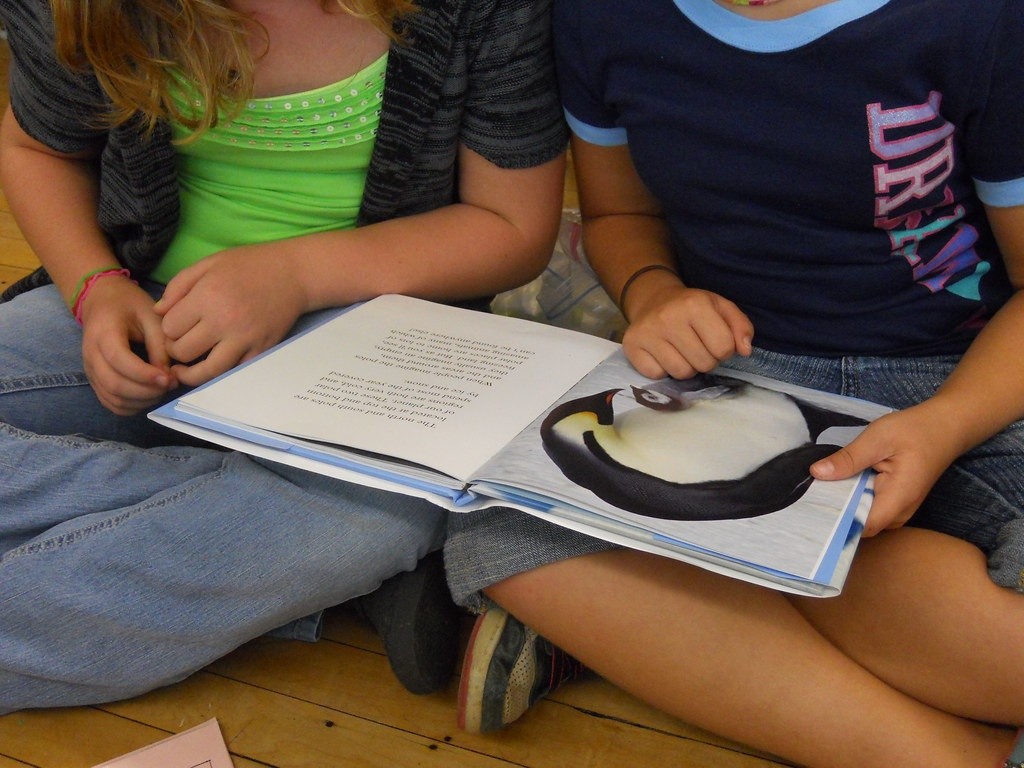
xmin=721 ymin=0 xmax=789 ymax=8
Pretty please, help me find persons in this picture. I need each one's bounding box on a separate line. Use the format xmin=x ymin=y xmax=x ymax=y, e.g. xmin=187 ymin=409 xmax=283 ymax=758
xmin=443 ymin=0 xmax=1024 ymax=768
xmin=1 ymin=0 xmax=579 ymax=719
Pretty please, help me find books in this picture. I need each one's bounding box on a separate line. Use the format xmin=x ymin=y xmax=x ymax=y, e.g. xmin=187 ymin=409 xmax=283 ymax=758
xmin=147 ymin=293 xmax=897 ymax=600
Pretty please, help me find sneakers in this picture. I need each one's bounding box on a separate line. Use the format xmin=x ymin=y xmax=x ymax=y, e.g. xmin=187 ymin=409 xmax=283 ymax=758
xmin=454 ymin=603 xmax=599 ymax=741
xmin=351 ymin=557 xmax=472 ymax=693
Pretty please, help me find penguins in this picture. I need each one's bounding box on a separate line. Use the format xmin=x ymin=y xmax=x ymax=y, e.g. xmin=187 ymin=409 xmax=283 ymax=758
xmin=538 ymin=378 xmax=876 ymax=522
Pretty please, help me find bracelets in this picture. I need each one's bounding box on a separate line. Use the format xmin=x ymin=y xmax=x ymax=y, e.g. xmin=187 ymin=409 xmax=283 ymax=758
xmin=621 ymin=265 xmax=679 ymax=323
xmin=71 ymin=265 xmax=139 ymax=328
